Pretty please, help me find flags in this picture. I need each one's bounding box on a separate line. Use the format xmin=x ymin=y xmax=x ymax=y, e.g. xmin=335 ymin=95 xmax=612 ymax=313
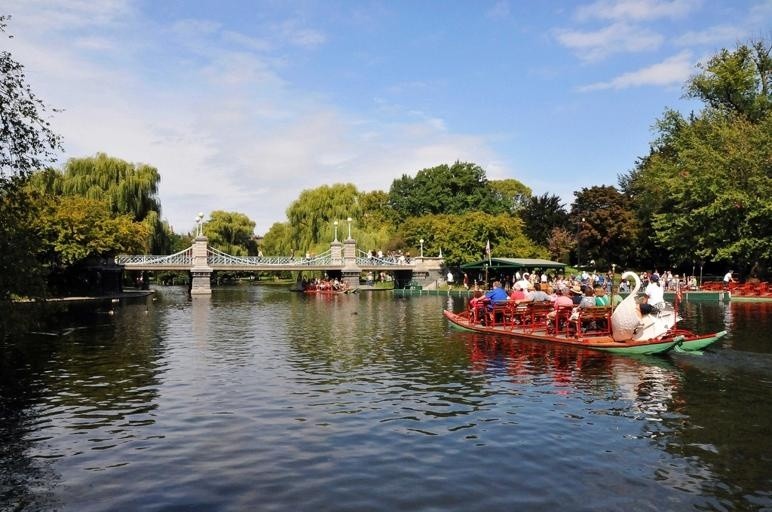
xmin=486 ymin=240 xmax=489 ymax=254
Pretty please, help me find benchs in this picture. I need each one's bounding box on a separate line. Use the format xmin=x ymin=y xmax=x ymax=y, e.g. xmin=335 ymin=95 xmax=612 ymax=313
xmin=305 ymin=280 xmax=346 ymax=291
xmin=682 ymin=280 xmax=772 ymax=298
xmin=468 ymin=299 xmax=618 ymax=338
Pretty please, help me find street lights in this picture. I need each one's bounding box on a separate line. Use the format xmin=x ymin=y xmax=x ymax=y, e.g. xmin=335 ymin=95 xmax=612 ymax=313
xmin=333 ymin=221 xmax=338 ymax=242
xmin=420 ymin=239 xmax=425 ymax=258
xmin=195 ymin=211 xmax=204 ymax=237
xmin=571 ymin=203 xmax=586 ymax=271
xmin=347 ymin=217 xmax=352 ymax=240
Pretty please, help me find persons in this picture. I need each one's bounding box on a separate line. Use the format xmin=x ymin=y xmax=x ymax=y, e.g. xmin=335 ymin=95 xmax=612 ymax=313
xmin=446 ymin=269 xmax=454 ymax=288
xmin=723 ymin=269 xmax=736 ymax=290
xmin=257 ymin=247 xmax=263 ymax=262
xmin=461 ymin=268 xmax=698 ymax=335
xmin=366 ymin=248 xmax=385 ymax=285
xmin=303 ymin=272 xmax=339 ymax=291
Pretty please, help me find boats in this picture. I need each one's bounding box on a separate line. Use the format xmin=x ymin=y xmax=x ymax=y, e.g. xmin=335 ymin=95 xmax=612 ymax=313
xmin=443 ymin=297 xmax=728 ymax=356
xmin=303 ymin=282 xmax=359 ymax=295
xmin=618 ymin=278 xmax=772 ymax=302
